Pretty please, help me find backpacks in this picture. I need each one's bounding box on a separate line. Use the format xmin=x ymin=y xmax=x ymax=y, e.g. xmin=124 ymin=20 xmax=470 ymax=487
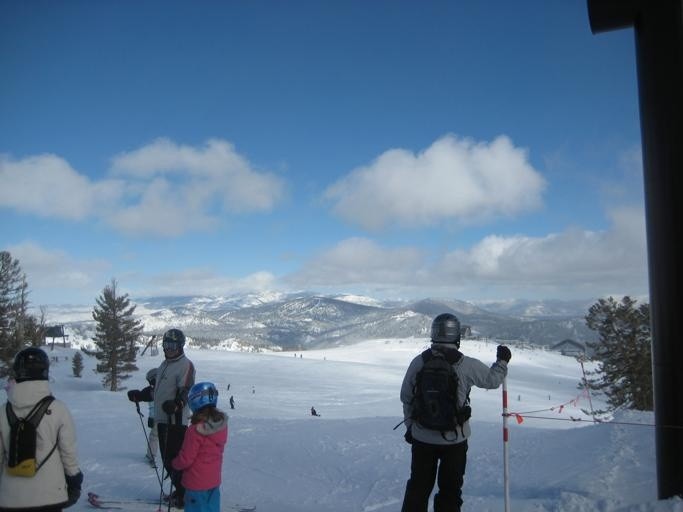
xmin=4 ymin=396 xmax=59 ymax=480
xmin=409 ymin=348 xmax=473 ymax=433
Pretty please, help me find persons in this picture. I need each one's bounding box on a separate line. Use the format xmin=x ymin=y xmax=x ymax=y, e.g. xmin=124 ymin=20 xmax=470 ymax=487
xmin=146 ymin=368 xmax=159 ymax=463
xmin=400 ymin=312 xmax=511 ymax=512
xmin=0 ymin=347 xmax=84 ymax=512
xmin=230 ymin=396 xmax=235 ymax=409
xmin=126 ymin=329 xmax=196 ymax=509
xmin=311 ymin=407 xmax=320 ymax=417
xmin=171 ymin=381 xmax=228 ymax=512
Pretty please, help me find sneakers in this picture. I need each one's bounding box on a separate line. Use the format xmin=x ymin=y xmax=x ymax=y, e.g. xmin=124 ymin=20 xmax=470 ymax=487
xmin=168 ymin=491 xmax=186 ymax=510
xmin=145 ymin=452 xmax=159 ymax=469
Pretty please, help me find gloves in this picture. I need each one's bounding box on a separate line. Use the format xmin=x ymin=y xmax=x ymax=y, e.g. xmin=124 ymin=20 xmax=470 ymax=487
xmin=162 ymin=392 xmax=187 ymax=417
xmin=62 ymin=471 xmax=83 ymax=509
xmin=496 ymin=344 xmax=512 ymax=363
xmin=166 ymin=459 xmax=176 ymax=473
xmin=404 ymin=427 xmax=415 ymax=445
xmin=127 ymin=387 xmax=153 ymax=402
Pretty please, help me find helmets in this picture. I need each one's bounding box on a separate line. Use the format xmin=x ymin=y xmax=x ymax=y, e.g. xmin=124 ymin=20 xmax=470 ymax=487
xmin=429 ymin=313 xmax=462 ymax=345
xmin=145 ymin=368 xmax=161 ymax=385
xmin=12 ymin=347 xmax=51 ymax=383
xmin=162 ymin=329 xmax=186 ymax=353
xmin=187 ymin=381 xmax=218 ymax=414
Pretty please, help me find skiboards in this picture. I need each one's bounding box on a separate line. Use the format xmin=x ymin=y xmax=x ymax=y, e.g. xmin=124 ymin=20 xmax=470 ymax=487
xmin=86 ymin=491 xmax=257 ymax=511
